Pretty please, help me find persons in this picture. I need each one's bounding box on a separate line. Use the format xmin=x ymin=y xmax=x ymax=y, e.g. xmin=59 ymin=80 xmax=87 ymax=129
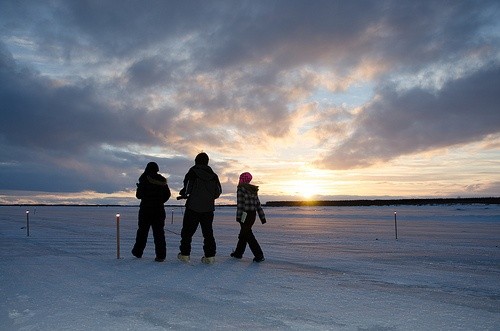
xmin=131 ymin=161 xmax=171 ymax=262
xmin=230 ymin=172 xmax=267 ymax=262
xmin=177 ymin=153 xmax=222 ymax=264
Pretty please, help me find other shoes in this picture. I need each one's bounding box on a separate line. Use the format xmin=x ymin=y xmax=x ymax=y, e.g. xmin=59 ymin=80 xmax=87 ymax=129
xmin=177 ymin=253 xmax=190 ymax=262
xmin=155 ymin=257 xmax=164 ymax=262
xmin=252 ymin=257 xmax=265 ymax=263
xmin=230 ymin=253 xmax=242 ymax=259
xmin=201 ymin=256 xmax=215 ymax=265
xmin=132 ymin=250 xmax=141 ymax=258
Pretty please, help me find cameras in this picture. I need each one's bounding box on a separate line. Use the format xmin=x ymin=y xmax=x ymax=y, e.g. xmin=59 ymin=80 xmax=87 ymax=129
xmin=177 ymin=194 xmax=189 ymax=200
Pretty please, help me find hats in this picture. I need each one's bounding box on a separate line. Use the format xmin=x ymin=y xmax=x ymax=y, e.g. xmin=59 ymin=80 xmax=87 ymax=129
xmin=194 ymin=152 xmax=209 ymax=164
xmin=146 ymin=162 xmax=159 ymax=172
xmin=238 ymin=172 xmax=252 ymax=184
